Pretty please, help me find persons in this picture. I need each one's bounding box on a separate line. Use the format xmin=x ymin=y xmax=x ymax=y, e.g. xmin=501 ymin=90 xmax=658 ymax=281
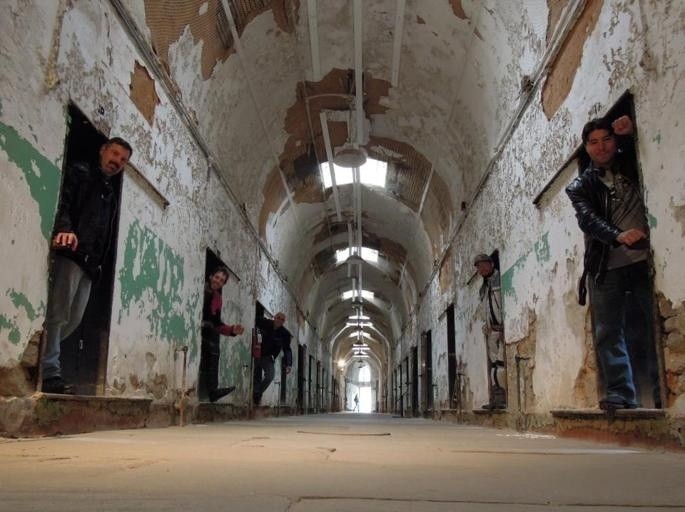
xmin=469 ymin=253 xmax=509 ymax=413
xmin=199 ymin=265 xmax=245 ymax=403
xmin=39 ymin=134 xmax=135 ymax=394
xmin=253 ymin=311 xmax=294 ymax=405
xmin=564 ymin=112 xmax=664 ymax=416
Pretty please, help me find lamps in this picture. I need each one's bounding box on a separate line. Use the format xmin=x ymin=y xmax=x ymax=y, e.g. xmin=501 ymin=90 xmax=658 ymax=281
xmin=332 ymin=137 xmax=373 ymax=371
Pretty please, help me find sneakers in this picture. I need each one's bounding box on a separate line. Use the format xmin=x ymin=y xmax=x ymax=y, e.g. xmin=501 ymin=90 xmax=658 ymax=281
xmin=209 ymin=386 xmax=236 ymax=403
xmin=599 ymin=394 xmax=638 ymax=410
xmin=42 ymin=377 xmax=80 ymax=395
xmin=482 ymin=400 xmax=505 ymax=410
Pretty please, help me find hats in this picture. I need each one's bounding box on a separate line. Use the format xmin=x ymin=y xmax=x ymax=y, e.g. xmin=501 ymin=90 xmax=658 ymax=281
xmin=474 ymin=254 xmax=492 ymax=266
